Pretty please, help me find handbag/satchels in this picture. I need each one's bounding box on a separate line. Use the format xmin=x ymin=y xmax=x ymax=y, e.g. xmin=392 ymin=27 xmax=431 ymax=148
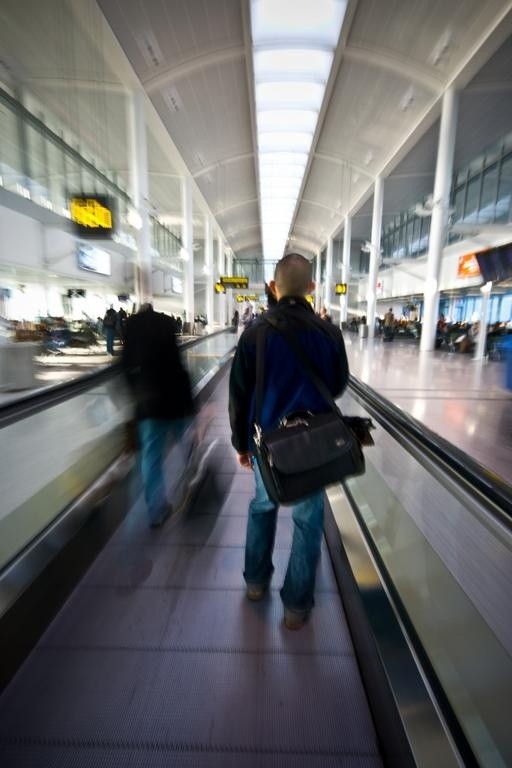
xmin=256 ymin=409 xmax=365 ymax=508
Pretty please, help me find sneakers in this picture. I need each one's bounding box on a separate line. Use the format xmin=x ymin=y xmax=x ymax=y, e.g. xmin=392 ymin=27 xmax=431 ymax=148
xmin=246 ymin=581 xmax=270 ymax=602
xmin=149 ymin=502 xmax=171 ymax=529
xmin=285 ymin=606 xmax=311 ymax=630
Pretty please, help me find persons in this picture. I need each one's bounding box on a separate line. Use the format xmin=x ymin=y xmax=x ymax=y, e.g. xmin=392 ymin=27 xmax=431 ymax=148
xmin=120 ymin=304 xmax=211 ymax=531
xmin=229 ymin=252 xmax=354 ymax=631
xmin=38 ymin=303 xmax=265 ymax=356
xmin=315 ymin=302 xmax=512 ymax=359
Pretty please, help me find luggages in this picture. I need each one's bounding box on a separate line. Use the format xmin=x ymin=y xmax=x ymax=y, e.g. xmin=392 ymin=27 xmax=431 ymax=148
xmin=381 ymin=326 xmax=393 ymax=342
